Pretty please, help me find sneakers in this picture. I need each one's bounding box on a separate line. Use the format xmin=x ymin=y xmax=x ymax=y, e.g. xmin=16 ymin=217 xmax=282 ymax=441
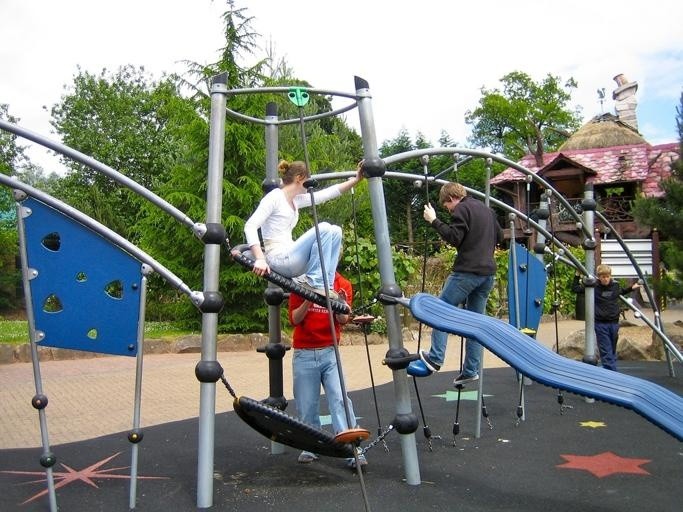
xmin=303 ymin=281 xmax=338 ymax=300
xmin=298 ymin=453 xmax=319 ymax=463
xmin=352 ymin=460 xmax=368 ymax=468
xmin=419 ymin=350 xmax=440 ymax=372
xmin=453 ymin=370 xmax=479 ymax=385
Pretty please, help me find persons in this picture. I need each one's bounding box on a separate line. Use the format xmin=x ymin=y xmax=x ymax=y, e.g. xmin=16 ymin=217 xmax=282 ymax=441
xmin=419 ymin=182 xmax=505 ymax=386
xmin=571 ymin=264 xmax=643 ymax=370
xmin=243 ymin=159 xmax=368 ymax=469
